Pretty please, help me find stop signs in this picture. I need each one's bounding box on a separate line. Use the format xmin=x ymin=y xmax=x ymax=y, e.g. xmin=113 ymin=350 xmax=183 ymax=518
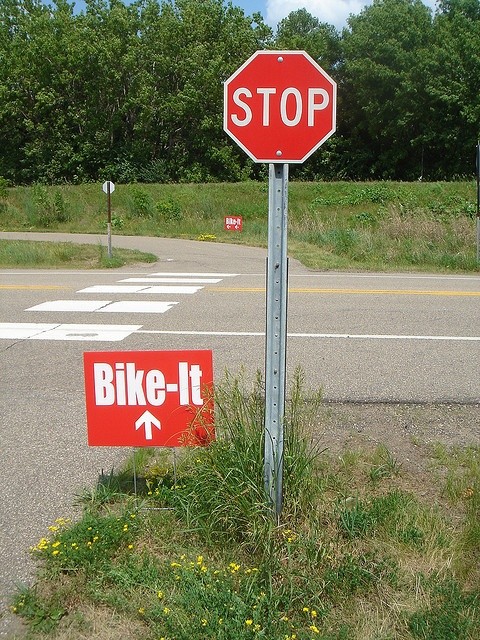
xmin=222 ymin=50 xmax=338 ymax=164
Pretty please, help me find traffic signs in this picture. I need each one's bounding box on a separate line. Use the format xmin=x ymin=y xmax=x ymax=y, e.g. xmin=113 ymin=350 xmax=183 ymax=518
xmin=83 ymin=349 xmax=216 ymax=448
xmin=224 ymin=215 xmax=242 ymax=231
